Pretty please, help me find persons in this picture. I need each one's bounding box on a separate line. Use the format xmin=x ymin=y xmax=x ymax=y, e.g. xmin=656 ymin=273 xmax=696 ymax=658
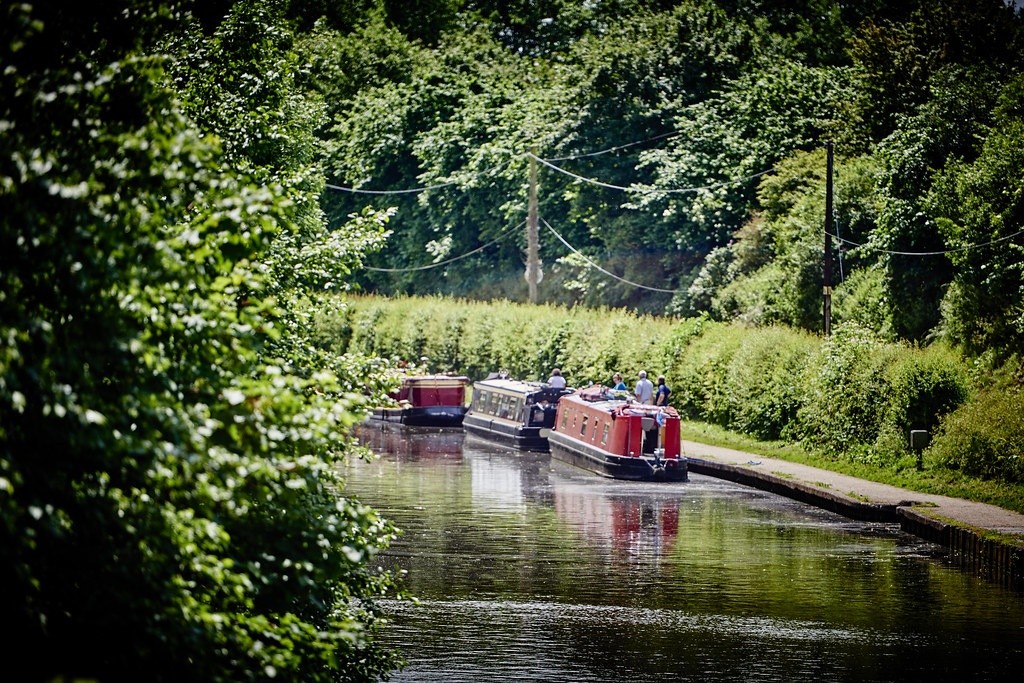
xmin=397 ymin=356 xmax=422 ymax=369
xmin=612 ymin=370 xmax=671 ymax=406
xmin=547 ymin=368 xmax=566 ymax=388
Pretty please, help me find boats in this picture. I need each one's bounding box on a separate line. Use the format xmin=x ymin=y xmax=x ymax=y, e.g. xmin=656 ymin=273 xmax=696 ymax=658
xmin=390 ymin=375 xmax=471 ymax=426
xmin=541 ymin=389 xmax=690 ymax=484
xmin=462 ymin=378 xmax=578 ymax=452
